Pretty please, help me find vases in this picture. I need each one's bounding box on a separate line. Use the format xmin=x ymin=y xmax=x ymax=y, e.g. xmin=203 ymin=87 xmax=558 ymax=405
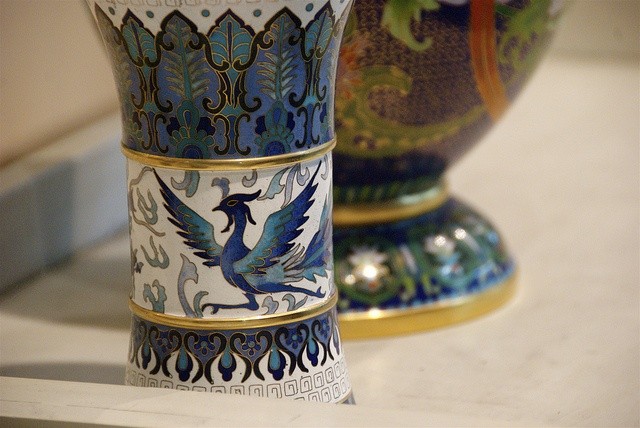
xmin=331 ymin=0 xmax=569 ymax=339
xmin=81 ymin=0 xmax=364 ymax=408
